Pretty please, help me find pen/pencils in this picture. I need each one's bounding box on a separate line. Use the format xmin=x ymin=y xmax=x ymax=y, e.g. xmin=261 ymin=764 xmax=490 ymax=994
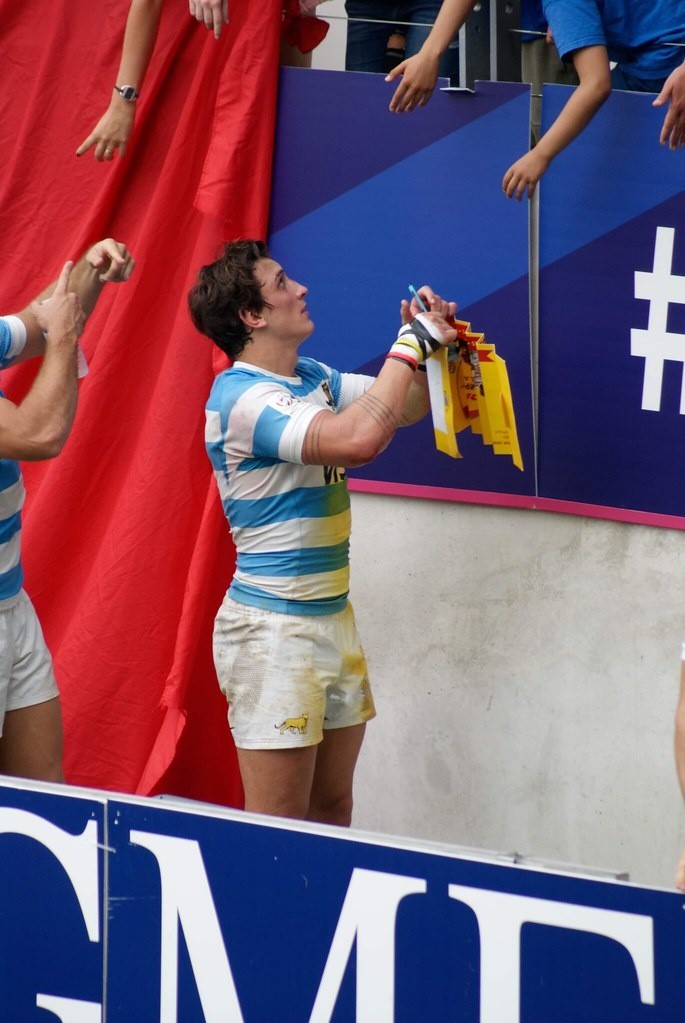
xmin=408 ymin=285 xmax=428 ymax=315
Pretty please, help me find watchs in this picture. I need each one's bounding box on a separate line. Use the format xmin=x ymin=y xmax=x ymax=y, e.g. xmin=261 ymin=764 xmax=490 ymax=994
xmin=112 ymin=83 xmax=141 ymax=103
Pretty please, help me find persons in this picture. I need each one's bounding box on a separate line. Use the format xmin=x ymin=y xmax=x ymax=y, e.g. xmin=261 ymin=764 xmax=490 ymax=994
xmin=0 ymin=237 xmax=135 ymax=784
xmin=185 ymin=238 xmax=458 ymax=831
xmin=74 ymin=0 xmax=163 ymax=163
xmin=188 ymin=0 xmax=329 ymax=69
xmin=342 ymin=0 xmax=685 ymax=202
